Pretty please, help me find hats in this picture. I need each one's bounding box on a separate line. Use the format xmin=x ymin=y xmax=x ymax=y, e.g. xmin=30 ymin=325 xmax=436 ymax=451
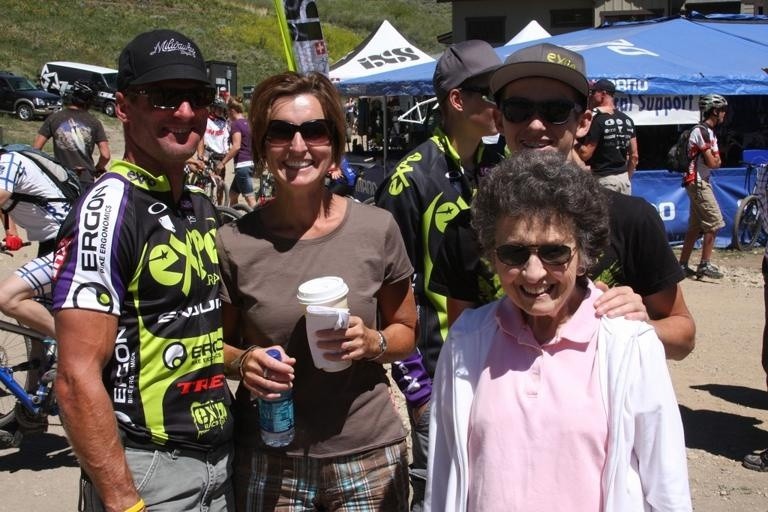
xmin=488 ymin=43 xmax=589 ymax=95
xmin=432 ymin=39 xmax=503 ymax=94
xmin=588 ymin=77 xmax=617 ymax=94
xmin=115 ymin=27 xmax=214 ymax=86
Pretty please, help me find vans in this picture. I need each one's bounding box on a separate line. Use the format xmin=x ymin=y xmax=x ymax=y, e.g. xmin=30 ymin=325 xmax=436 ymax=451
xmin=36 ymin=58 xmax=118 ymax=118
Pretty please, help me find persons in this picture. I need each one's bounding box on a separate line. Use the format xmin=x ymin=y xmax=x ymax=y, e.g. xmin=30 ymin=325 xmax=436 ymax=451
xmin=680 ymin=94 xmax=726 ymax=281
xmin=743 ymin=255 xmax=768 ymax=472
xmin=0 ymin=146 xmax=81 ymax=427
xmin=49 ymin=31 xmax=694 ymax=511
xmin=36 ymin=91 xmax=110 ymax=194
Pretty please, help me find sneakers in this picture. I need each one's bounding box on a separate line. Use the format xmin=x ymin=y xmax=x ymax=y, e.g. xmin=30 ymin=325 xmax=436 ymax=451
xmin=2 ymin=405 xmax=51 ymax=447
xmin=739 ymin=441 xmax=768 ymax=470
xmin=674 ymin=262 xmax=700 ymax=280
xmin=35 ymin=344 xmax=61 ymax=391
xmin=697 ymin=263 xmax=724 ymax=278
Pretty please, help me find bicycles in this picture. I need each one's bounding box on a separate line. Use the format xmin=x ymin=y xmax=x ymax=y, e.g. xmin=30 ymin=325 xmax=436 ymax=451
xmin=731 ymin=160 xmax=767 ymax=253
xmin=188 ymin=158 xmax=273 ymax=226
xmin=0 ymin=239 xmax=60 ymax=451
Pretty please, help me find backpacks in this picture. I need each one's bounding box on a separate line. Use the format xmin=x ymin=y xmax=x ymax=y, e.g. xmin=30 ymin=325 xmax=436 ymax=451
xmin=665 ymin=124 xmax=711 ymax=172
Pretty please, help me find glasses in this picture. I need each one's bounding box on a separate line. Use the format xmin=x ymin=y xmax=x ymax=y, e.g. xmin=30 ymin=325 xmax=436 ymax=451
xmin=124 ymin=83 xmax=222 ymax=111
xmin=493 ymin=239 xmax=572 ymax=266
xmin=500 ymin=96 xmax=588 ymax=124
xmin=256 ymin=116 xmax=335 ymax=147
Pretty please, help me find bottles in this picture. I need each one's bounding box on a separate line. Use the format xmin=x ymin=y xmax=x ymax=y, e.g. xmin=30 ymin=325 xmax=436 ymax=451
xmin=259 ymin=348 xmax=296 ymax=449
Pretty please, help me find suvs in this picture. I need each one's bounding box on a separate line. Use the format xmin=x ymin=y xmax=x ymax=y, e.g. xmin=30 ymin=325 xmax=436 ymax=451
xmin=0 ymin=67 xmax=65 ymax=122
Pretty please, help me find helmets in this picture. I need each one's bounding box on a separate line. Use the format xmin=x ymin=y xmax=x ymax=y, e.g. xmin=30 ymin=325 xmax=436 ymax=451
xmin=208 ymin=95 xmax=226 ymax=112
xmin=698 ymin=93 xmax=728 ymax=116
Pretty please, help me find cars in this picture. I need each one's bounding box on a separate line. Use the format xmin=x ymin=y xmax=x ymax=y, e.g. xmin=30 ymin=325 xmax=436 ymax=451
xmin=241 ymin=84 xmax=255 ymax=98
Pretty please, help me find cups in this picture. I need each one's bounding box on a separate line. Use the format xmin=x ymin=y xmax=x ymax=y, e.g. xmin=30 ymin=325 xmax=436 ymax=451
xmin=296 ymin=274 xmax=355 ymax=373
